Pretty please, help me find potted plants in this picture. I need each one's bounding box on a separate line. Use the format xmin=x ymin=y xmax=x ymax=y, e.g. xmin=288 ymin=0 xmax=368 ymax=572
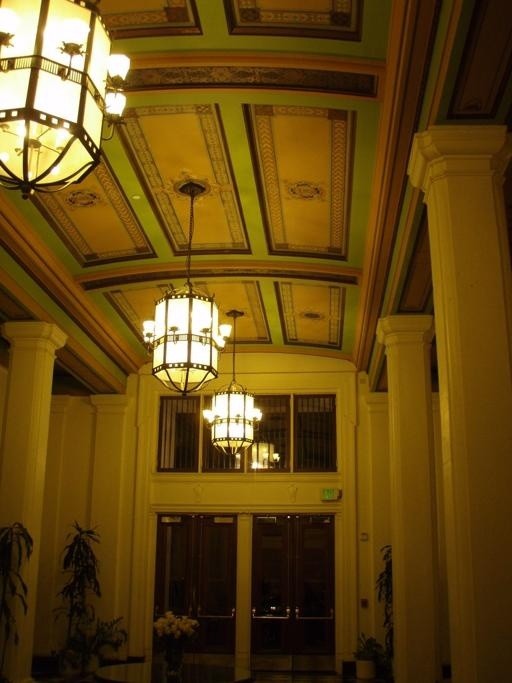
xmin=352 ymin=632 xmax=382 ymax=679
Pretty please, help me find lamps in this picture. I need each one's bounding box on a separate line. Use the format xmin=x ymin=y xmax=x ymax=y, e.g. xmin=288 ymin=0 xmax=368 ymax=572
xmin=140 ymin=191 xmax=233 ymax=397
xmin=200 ymin=317 xmax=255 ymax=457
xmin=250 ymin=441 xmax=276 ymax=469
xmin=1 ymin=0 xmax=131 ymax=201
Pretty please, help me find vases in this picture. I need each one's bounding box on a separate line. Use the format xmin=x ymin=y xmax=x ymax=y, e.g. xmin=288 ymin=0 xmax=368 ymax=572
xmin=165 ymin=664 xmax=182 ymax=683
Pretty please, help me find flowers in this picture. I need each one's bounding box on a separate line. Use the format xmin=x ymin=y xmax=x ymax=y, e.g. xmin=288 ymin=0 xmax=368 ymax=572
xmin=155 ymin=612 xmax=199 ymax=669
